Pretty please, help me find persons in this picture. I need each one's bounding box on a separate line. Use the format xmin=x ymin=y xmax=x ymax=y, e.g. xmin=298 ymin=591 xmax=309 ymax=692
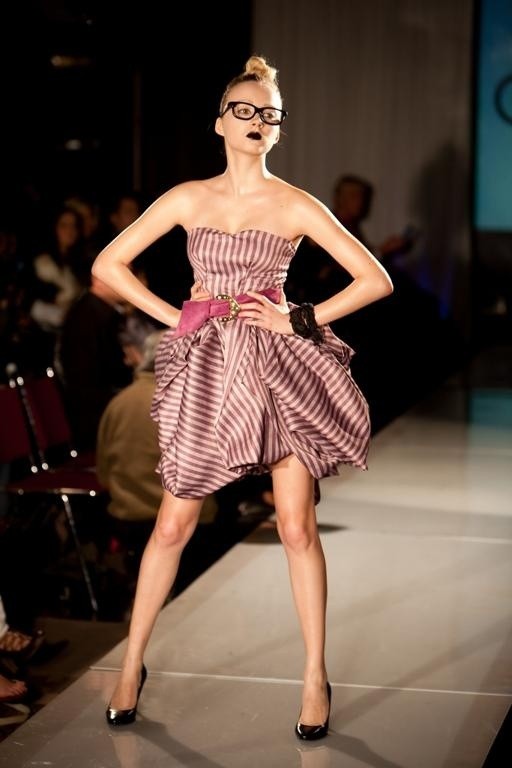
xmin=298 ymin=174 xmax=374 ymax=305
xmin=60 ymin=233 xmax=145 ymax=455
xmin=86 ymin=54 xmax=396 ymax=742
xmin=14 ymin=194 xmax=151 ymax=338
xmin=97 ymin=329 xmax=217 ymax=601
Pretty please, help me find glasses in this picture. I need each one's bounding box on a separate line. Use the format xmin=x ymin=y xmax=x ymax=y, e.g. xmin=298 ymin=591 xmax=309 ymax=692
xmin=220 ymin=101 xmax=289 ymax=125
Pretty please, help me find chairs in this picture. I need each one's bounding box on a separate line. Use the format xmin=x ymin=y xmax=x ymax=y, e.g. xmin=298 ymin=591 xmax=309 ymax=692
xmin=0 ymin=371 xmax=108 ymax=613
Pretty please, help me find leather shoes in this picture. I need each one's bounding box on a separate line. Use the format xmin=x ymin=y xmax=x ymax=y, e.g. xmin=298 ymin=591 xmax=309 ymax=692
xmin=295 ymin=682 xmax=332 ymax=740
xmin=106 ymin=664 xmax=147 ymax=726
